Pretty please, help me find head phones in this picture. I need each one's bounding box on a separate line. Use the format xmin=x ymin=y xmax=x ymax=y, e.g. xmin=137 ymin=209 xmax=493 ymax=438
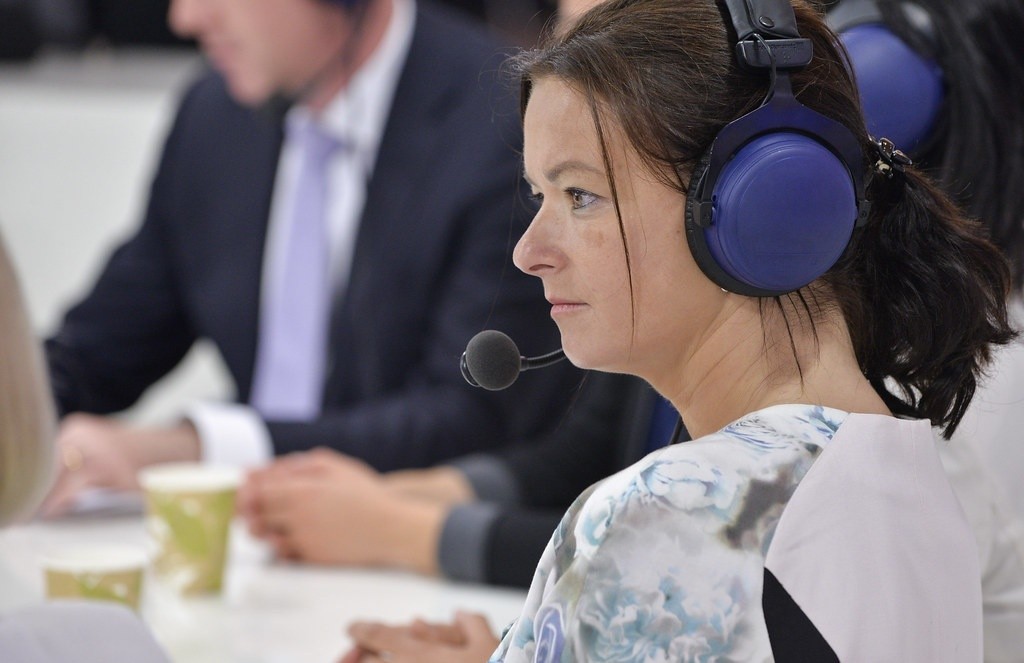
xmin=682 ymin=0 xmax=871 ymax=305
xmin=814 ymin=0 xmax=955 ymax=168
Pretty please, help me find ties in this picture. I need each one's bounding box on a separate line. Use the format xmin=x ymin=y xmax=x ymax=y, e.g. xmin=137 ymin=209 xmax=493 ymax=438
xmin=247 ymin=123 xmax=353 ymax=424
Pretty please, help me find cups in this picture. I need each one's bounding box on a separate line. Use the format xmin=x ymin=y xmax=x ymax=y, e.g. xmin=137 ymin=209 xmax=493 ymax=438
xmin=41 ymin=552 xmax=141 ymax=606
xmin=137 ymin=463 xmax=237 ymax=596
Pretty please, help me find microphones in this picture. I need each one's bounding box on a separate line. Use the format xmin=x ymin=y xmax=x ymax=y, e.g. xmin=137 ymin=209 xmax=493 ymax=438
xmin=258 ymin=13 xmax=372 ymax=121
xmin=460 ymin=331 xmax=564 ymax=390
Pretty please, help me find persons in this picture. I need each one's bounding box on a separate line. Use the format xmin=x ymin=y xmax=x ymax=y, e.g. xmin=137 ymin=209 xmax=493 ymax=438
xmin=337 ymin=0 xmax=1017 ymax=662
xmin=642 ymin=1 xmax=1024 ymax=662
xmin=25 ymin=0 xmax=580 ymax=527
xmin=234 ymin=0 xmax=769 ymax=593
